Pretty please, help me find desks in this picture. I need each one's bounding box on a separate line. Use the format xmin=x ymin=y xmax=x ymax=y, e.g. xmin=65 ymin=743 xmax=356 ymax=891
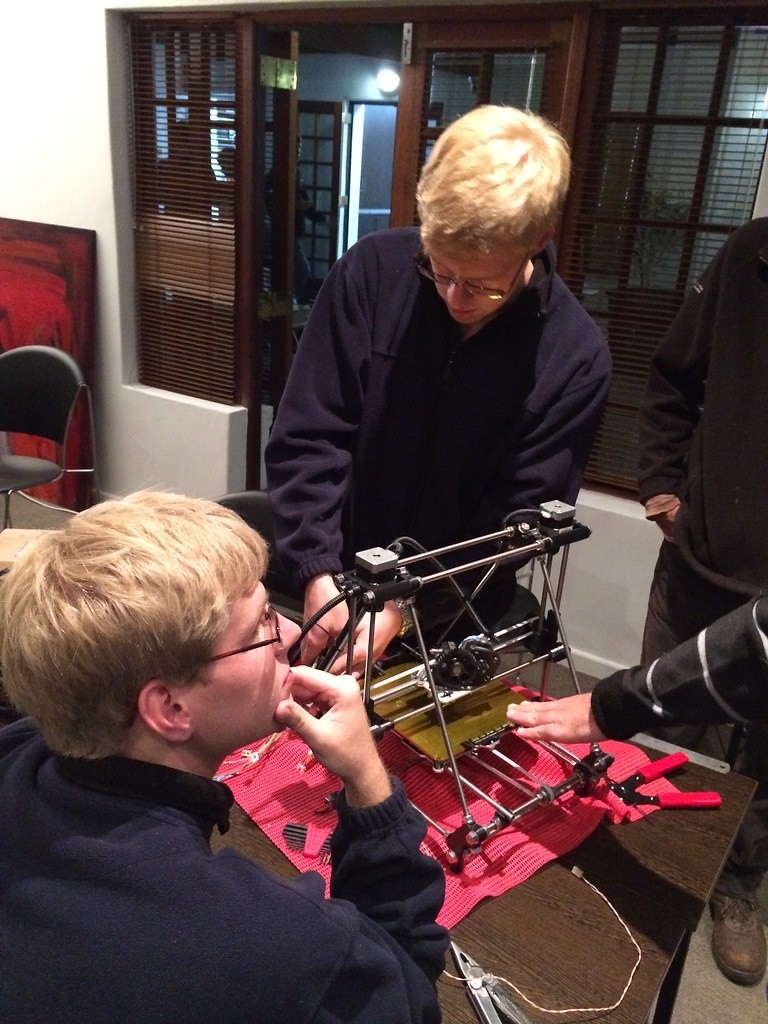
xmin=203 ymin=651 xmax=761 ymax=1024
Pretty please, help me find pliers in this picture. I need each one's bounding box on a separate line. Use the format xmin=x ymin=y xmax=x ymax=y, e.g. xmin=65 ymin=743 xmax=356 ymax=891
xmin=448 ymin=940 xmax=533 ymax=1024
xmin=604 ymin=751 xmax=723 ymax=811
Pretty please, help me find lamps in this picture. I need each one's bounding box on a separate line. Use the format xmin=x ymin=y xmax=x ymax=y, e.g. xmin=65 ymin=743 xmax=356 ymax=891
xmin=377 ymin=69 xmax=400 ymax=93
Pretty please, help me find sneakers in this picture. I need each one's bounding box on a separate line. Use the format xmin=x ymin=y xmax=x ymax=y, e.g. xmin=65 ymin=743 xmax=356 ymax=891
xmin=710 ymin=888 xmax=766 ymax=985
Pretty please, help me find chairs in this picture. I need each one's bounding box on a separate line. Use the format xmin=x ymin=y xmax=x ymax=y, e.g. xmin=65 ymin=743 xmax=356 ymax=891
xmin=0 ymin=345 xmax=98 ymax=528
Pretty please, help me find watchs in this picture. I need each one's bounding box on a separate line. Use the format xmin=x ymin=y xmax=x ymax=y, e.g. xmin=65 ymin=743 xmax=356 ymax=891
xmin=392 ymin=596 xmax=417 ymax=638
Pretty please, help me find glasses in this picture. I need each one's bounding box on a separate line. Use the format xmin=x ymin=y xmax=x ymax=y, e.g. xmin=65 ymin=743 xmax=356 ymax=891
xmin=417 ymin=247 xmax=528 ymax=300
xmin=123 ymin=603 xmax=282 ymax=729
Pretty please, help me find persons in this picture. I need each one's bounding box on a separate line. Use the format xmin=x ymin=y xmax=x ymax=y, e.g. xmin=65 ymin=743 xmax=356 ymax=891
xmin=264 ymin=105 xmax=612 ymax=681
xmin=635 ymin=214 xmax=768 ymax=985
xmin=504 ymin=596 xmax=768 ymax=744
xmin=265 ymin=132 xmax=331 ymax=299
xmin=0 ymin=488 xmax=451 ymax=1024
